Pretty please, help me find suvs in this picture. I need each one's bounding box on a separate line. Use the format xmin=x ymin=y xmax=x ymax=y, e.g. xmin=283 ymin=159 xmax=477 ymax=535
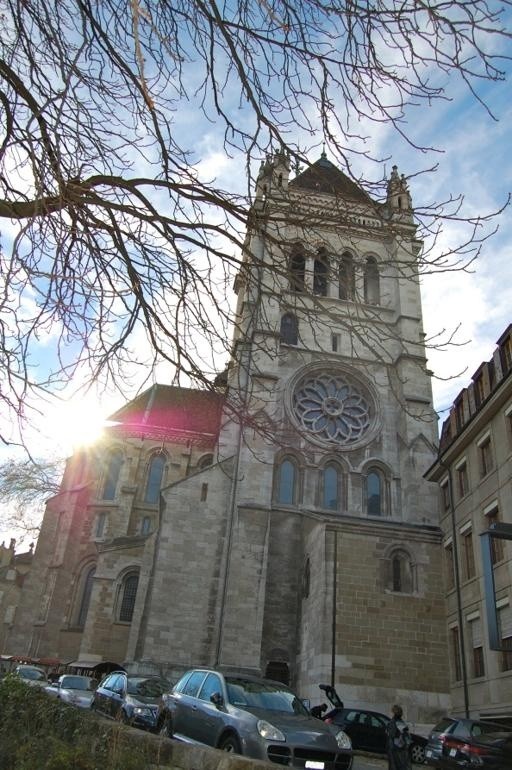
xmin=423 ymin=717 xmax=512 ymax=770
xmin=90 ymin=670 xmax=174 ymax=733
xmin=319 ymin=683 xmax=428 ymax=764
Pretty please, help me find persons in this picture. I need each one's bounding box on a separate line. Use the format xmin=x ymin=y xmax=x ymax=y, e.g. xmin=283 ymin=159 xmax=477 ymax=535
xmin=47 ymin=668 xmax=60 ymax=682
xmin=384 ymin=704 xmax=414 ymax=770
xmin=309 ymin=702 xmax=328 ymax=719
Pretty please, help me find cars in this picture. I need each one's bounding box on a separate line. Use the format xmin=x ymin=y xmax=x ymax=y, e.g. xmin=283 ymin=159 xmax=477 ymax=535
xmin=43 ymin=673 xmax=95 ymax=709
xmin=155 ymin=667 xmax=353 ymax=770
xmin=2 ymin=664 xmax=52 ymax=693
